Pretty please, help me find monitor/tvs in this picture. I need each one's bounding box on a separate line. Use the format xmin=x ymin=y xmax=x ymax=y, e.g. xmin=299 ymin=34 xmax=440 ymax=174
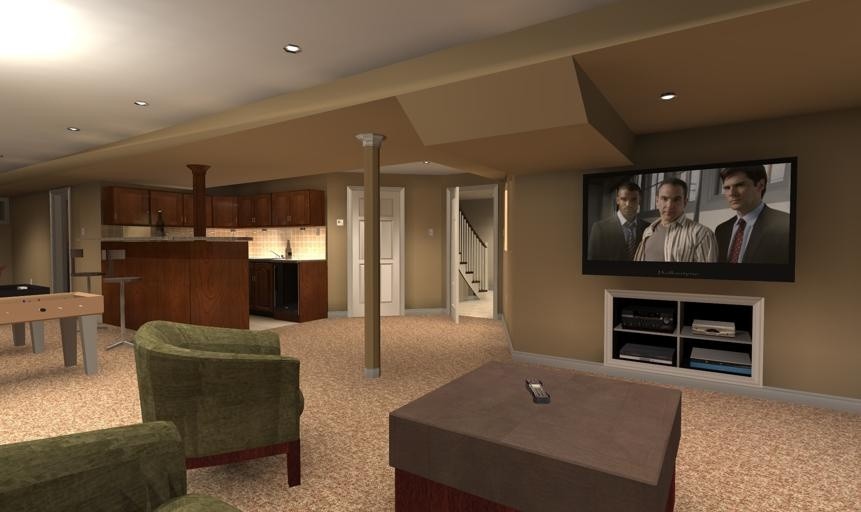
xmin=582 ymin=156 xmax=798 ymax=283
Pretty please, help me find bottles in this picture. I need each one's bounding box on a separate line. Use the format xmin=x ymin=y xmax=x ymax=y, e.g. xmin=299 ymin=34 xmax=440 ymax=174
xmin=285 ymin=239 xmax=292 ymax=261
xmin=155 ymin=210 xmax=165 ymax=237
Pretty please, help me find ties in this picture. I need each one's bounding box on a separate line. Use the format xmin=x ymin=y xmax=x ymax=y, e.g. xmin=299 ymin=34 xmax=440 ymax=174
xmin=625 ymin=222 xmax=637 ymax=258
xmin=730 ymin=219 xmax=747 ymax=264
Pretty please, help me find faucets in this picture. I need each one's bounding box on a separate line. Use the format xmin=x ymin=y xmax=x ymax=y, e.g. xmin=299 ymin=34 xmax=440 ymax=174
xmin=270 ymin=249 xmax=284 ymax=259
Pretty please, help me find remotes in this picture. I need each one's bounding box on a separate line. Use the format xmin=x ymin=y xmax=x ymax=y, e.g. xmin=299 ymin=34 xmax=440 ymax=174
xmin=525 ymin=376 xmax=551 ymax=404
